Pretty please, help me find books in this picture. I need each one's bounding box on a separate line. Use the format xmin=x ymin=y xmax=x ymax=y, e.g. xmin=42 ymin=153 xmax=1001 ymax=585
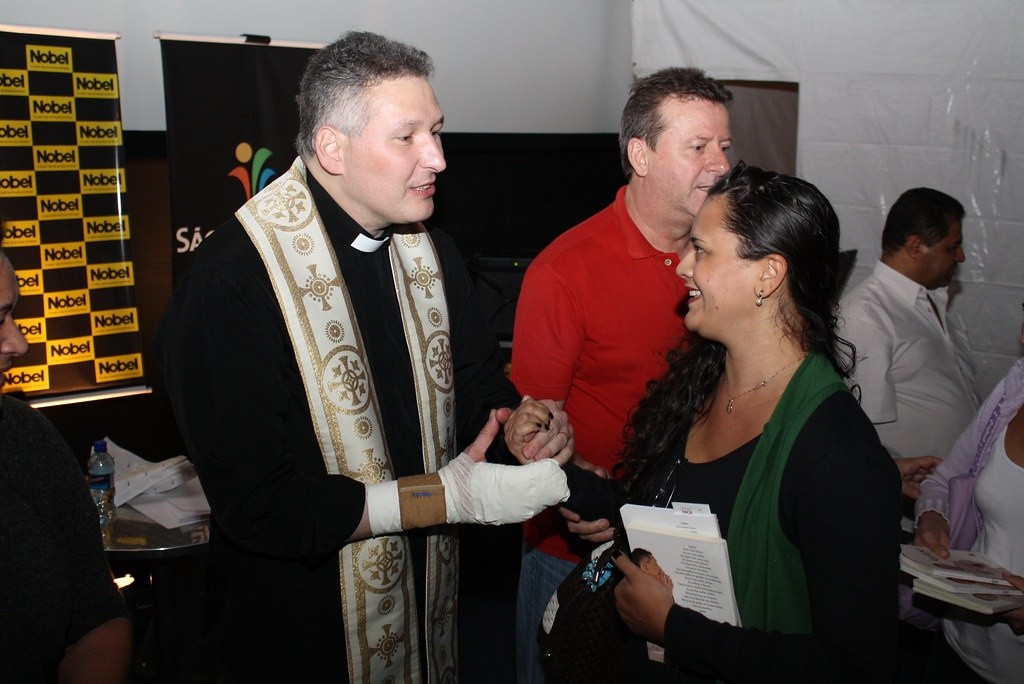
xmin=899 ymin=544 xmax=1022 ymax=614
xmin=620 ymin=504 xmax=742 ymax=663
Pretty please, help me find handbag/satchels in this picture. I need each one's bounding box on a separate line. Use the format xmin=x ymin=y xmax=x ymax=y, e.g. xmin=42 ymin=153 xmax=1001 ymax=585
xmin=896 ymin=390 xmax=1009 ymax=632
xmin=537 ymin=441 xmax=684 ymax=684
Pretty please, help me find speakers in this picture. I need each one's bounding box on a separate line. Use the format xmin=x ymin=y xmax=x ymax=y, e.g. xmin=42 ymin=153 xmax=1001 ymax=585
xmin=478 ymin=257 xmax=534 ymax=340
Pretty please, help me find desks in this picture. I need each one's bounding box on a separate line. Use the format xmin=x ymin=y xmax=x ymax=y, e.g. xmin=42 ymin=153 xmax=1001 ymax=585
xmin=82 ymin=472 xmax=210 ymax=604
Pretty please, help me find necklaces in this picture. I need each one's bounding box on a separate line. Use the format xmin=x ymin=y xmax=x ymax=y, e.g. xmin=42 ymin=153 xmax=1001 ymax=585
xmin=723 ymin=355 xmax=806 ymax=413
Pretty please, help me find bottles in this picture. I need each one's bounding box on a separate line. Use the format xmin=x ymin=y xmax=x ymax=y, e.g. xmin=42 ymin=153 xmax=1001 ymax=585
xmin=87 ymin=440 xmax=117 ymax=527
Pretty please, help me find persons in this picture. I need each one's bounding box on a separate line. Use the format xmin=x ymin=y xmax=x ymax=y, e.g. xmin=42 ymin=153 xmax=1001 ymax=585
xmin=912 ymin=298 xmax=1024 ymax=684
xmin=508 ymin=66 xmax=944 ymax=684
xmin=169 ymin=33 xmax=571 ymax=684
xmin=0 ymin=228 xmax=134 ymax=684
xmin=830 ymin=186 xmax=982 ymax=531
xmin=631 ymin=549 xmax=673 ymax=661
xmin=502 ymin=161 xmax=897 ymax=684
